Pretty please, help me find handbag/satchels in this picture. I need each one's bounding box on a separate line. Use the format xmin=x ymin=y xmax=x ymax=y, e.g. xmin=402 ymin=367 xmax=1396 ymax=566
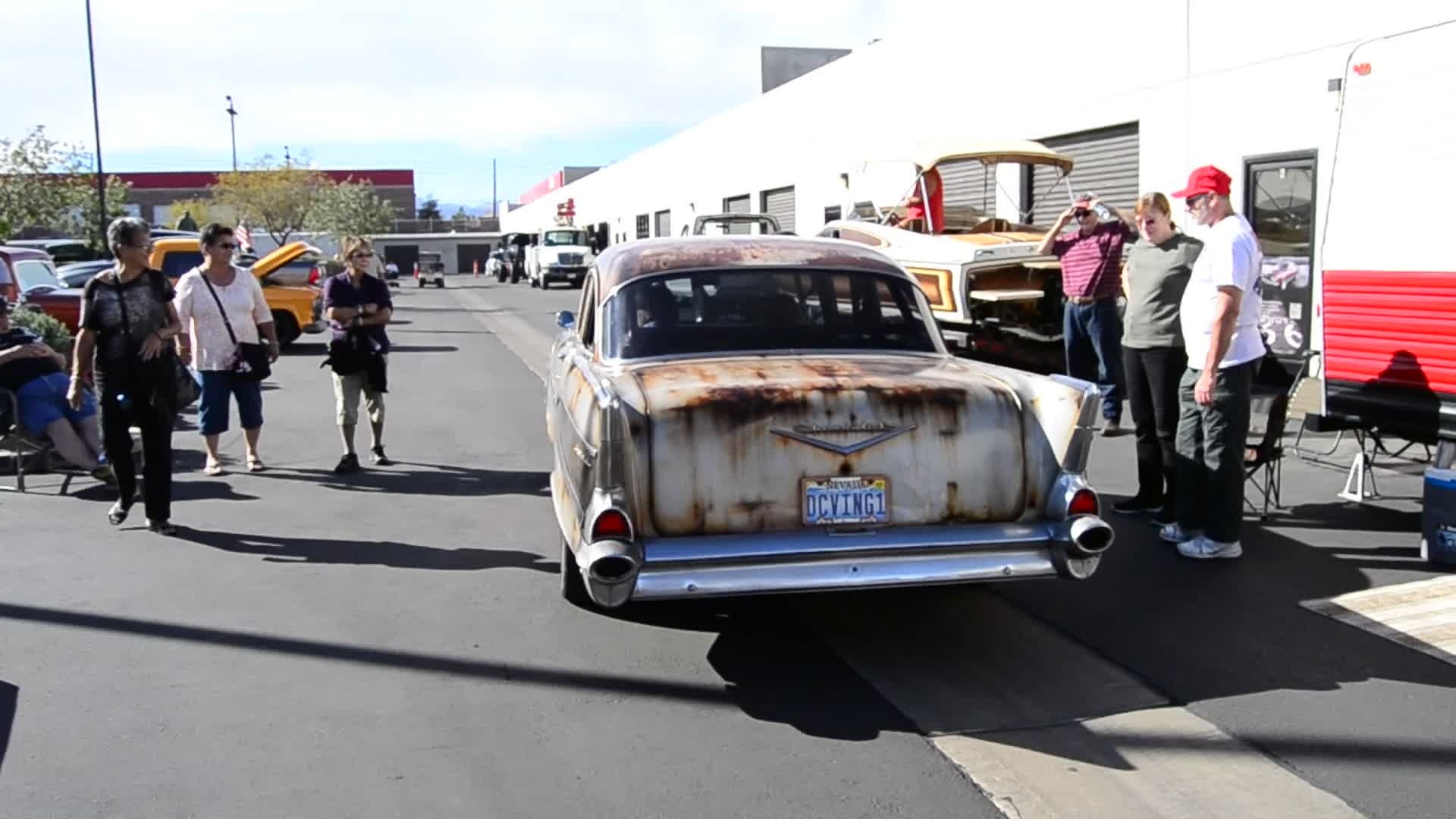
xmin=227 ymin=340 xmax=271 ymax=381
xmin=171 ymin=351 xmax=201 ymax=410
xmin=320 ymin=338 xmax=389 ymax=395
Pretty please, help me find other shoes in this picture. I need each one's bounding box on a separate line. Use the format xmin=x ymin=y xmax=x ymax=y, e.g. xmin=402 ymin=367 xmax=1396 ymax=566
xmin=143 ymin=516 xmax=177 ymax=536
xmin=203 ymin=461 xmax=222 ymax=475
xmin=108 ymin=499 xmax=132 ymax=524
xmin=246 ymin=454 xmax=265 ymax=472
xmin=370 ymin=444 xmax=392 ymax=465
xmin=333 ymin=451 xmax=359 ymax=473
xmin=1100 ymin=418 xmax=1117 ymax=436
xmin=92 ymin=460 xmax=141 ymax=498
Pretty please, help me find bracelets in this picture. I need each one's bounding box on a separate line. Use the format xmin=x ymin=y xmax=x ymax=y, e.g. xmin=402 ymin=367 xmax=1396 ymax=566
xmin=68 ymin=375 xmax=82 ymax=381
xmin=358 ymin=317 xmax=364 ymax=327
xmin=176 ymin=345 xmax=189 ymax=349
xmin=265 ymin=339 xmax=279 ymax=346
xmin=153 ymin=330 xmax=164 ymax=341
xmin=357 ymin=303 xmax=364 ymax=316
xmin=907 ymin=197 xmax=912 ymax=204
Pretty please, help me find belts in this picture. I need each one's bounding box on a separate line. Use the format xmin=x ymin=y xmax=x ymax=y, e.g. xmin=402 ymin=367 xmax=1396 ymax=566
xmin=1068 ymin=293 xmax=1113 ymax=306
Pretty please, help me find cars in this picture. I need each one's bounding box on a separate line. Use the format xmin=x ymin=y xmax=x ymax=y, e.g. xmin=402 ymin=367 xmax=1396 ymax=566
xmin=1 ymin=230 xmax=203 ymax=337
xmin=148 ymin=237 xmax=327 ymax=350
xmin=545 ymin=234 xmax=1117 ymax=611
xmin=416 ymin=250 xmax=445 ymax=289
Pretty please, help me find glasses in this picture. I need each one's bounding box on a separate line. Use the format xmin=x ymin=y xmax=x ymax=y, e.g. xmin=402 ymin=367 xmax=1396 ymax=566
xmin=130 ymin=243 xmax=155 ymax=249
xmin=351 ymin=252 xmax=373 ymax=258
xmin=1074 ymin=210 xmax=1090 ymax=216
xmin=1134 ymin=216 xmax=1164 ymax=226
xmin=212 ymin=242 xmax=236 ymax=250
xmin=1184 ymin=193 xmax=1206 ymax=205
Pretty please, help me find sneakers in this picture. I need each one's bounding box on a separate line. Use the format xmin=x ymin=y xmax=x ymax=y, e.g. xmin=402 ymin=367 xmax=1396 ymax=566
xmin=1179 ymin=534 xmax=1244 ymax=559
xmin=1151 ymin=503 xmax=1180 ymax=525
xmin=1161 ymin=524 xmax=1190 ymax=542
xmin=1111 ymin=496 xmax=1164 ymax=514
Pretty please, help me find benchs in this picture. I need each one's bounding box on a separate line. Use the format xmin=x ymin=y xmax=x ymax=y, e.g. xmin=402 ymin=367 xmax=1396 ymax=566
xmin=621 ymin=321 xmax=939 ymax=359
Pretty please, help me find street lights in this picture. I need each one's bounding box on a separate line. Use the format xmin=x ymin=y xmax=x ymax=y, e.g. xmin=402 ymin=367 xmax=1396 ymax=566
xmin=225 ymin=95 xmax=237 ymax=172
xmin=284 ymin=145 xmax=291 ymax=169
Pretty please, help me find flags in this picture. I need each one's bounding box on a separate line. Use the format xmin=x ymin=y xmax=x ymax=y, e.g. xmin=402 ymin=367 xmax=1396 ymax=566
xmin=235 ymin=217 xmax=255 ymax=250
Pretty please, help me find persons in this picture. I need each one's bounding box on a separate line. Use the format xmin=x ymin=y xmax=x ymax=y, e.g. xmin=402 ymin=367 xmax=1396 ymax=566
xmin=1108 ymin=190 xmax=1205 ymax=528
xmin=1159 ymin=165 xmax=1269 ymax=562
xmin=1039 ymin=192 xmax=1138 ymax=436
xmin=0 ymin=293 xmax=144 ymax=485
xmin=322 ymin=234 xmax=397 ymax=474
xmin=170 ymin=221 xmax=279 ymax=476
xmin=901 ymin=161 xmax=947 ymax=235
xmin=175 ymin=209 xmax=198 ymax=231
xmin=624 ymin=283 xmax=686 ymax=346
xmin=65 ymin=215 xmax=187 ymax=539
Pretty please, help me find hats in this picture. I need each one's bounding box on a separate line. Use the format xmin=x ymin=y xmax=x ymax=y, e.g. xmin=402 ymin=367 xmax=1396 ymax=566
xmin=1170 ymin=165 xmax=1231 ymax=197
xmin=0 ymin=297 xmax=15 ymax=310
xmin=1071 ymin=194 xmax=1095 ymax=209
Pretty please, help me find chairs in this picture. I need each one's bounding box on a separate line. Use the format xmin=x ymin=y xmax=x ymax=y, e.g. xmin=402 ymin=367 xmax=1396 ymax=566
xmin=0 ymin=372 xmax=117 ymax=493
xmin=1243 ymin=350 xmax=1322 ymax=521
xmin=968 ymin=218 xmax=1013 ymax=234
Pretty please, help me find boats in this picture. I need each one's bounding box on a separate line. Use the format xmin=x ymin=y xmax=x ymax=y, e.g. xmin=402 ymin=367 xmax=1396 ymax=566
xmin=813 ymin=141 xmax=1130 ymax=366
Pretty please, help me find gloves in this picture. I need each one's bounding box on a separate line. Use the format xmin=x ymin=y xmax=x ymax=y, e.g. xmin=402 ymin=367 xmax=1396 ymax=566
xmin=1087 ymin=190 xmax=1116 ymax=217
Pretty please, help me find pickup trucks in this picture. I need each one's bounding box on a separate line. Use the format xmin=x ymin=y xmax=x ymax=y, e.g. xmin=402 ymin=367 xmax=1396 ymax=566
xmin=494 ymin=233 xmax=539 ymax=285
xmin=525 ymin=226 xmax=593 ymax=290
